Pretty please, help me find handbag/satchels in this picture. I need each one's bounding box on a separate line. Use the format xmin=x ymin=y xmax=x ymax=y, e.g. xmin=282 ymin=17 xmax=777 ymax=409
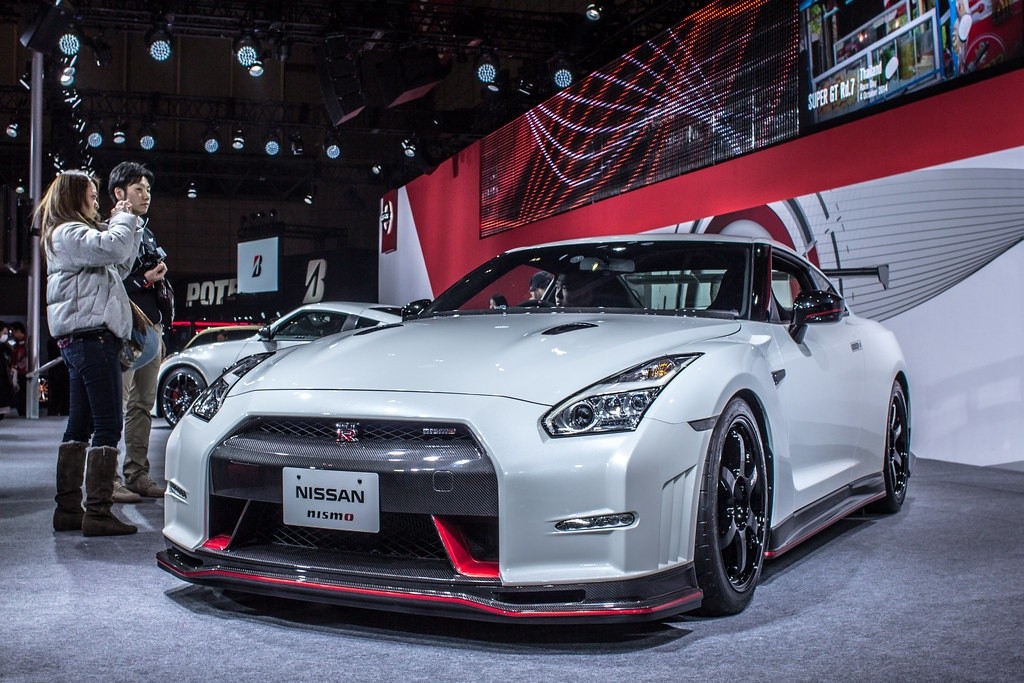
xmin=118 ymin=299 xmax=147 ymax=372
xmin=155 ymin=277 xmax=174 ymax=311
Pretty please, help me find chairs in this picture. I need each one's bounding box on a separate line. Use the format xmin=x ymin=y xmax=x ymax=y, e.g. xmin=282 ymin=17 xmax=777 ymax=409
xmin=564 ymin=268 xmax=643 ymax=308
xmin=705 ymin=264 xmax=745 ymax=312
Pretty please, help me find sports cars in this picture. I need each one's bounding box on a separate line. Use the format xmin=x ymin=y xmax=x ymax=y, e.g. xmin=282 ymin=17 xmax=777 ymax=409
xmin=155 ymin=232 xmax=913 ymax=625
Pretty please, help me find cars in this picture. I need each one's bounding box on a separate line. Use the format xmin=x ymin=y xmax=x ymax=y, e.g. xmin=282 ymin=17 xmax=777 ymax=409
xmin=35 ymin=301 xmax=406 ymax=430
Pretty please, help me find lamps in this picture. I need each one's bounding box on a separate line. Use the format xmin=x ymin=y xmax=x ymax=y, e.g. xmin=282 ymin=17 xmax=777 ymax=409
xmin=186 ymin=177 xmax=200 ymax=200
xmin=145 ymin=9 xmax=174 ymax=63
xmin=404 ymin=137 xmax=420 ymax=157
xmin=472 ymin=49 xmax=501 ymax=83
xmin=262 ymin=128 xmax=281 ymax=155
xmin=400 ymin=132 xmax=416 ymax=149
xmin=137 ymin=116 xmax=158 ymax=152
xmin=324 ymin=129 xmax=344 ymax=158
xmin=0 ymin=0 xmax=133 ymax=193
xmin=256 ymin=211 xmax=266 ymax=223
xmin=231 ymin=122 xmax=246 ymax=149
xmin=302 ymin=185 xmax=318 ymax=204
xmin=586 ymin=0 xmax=616 ymax=22
xmin=268 ymin=208 xmax=278 ymax=222
xmin=232 ymin=12 xmax=261 ymax=65
xmin=518 ymin=75 xmax=539 ymax=96
xmin=199 ymin=119 xmax=222 ymax=155
xmin=487 ymin=68 xmax=510 ymax=91
xmin=371 ymin=161 xmax=384 ymax=174
xmin=549 ymin=53 xmax=575 ymax=88
xmin=247 ymin=49 xmax=273 ymax=78
xmin=288 ymin=126 xmax=305 ymax=156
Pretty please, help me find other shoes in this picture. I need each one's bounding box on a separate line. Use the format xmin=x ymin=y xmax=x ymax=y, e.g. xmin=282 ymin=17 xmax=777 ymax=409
xmin=112 ymin=482 xmax=140 ymax=503
xmin=125 ymin=475 xmax=166 ymax=498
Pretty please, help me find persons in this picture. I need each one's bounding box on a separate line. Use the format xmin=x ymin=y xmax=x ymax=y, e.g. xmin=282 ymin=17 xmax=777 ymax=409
xmin=528 ymin=271 xmax=552 ymax=301
xmin=0 ymin=321 xmax=33 ymax=422
xmin=36 ymin=168 xmax=145 ymax=537
xmin=555 ymin=268 xmax=592 ymax=309
xmin=489 ymin=293 xmax=510 ymax=310
xmin=216 ymin=329 xmax=229 ymax=342
xmin=100 ymin=161 xmax=167 ymax=505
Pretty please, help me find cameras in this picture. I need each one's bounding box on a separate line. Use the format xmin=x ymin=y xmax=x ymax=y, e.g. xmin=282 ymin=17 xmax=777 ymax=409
xmin=139 ymin=246 xmax=168 ymax=270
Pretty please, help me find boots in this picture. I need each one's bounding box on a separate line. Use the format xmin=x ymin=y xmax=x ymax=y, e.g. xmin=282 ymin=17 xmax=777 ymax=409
xmin=53 ymin=440 xmax=90 ymax=532
xmin=82 ymin=444 xmax=138 ymax=536
